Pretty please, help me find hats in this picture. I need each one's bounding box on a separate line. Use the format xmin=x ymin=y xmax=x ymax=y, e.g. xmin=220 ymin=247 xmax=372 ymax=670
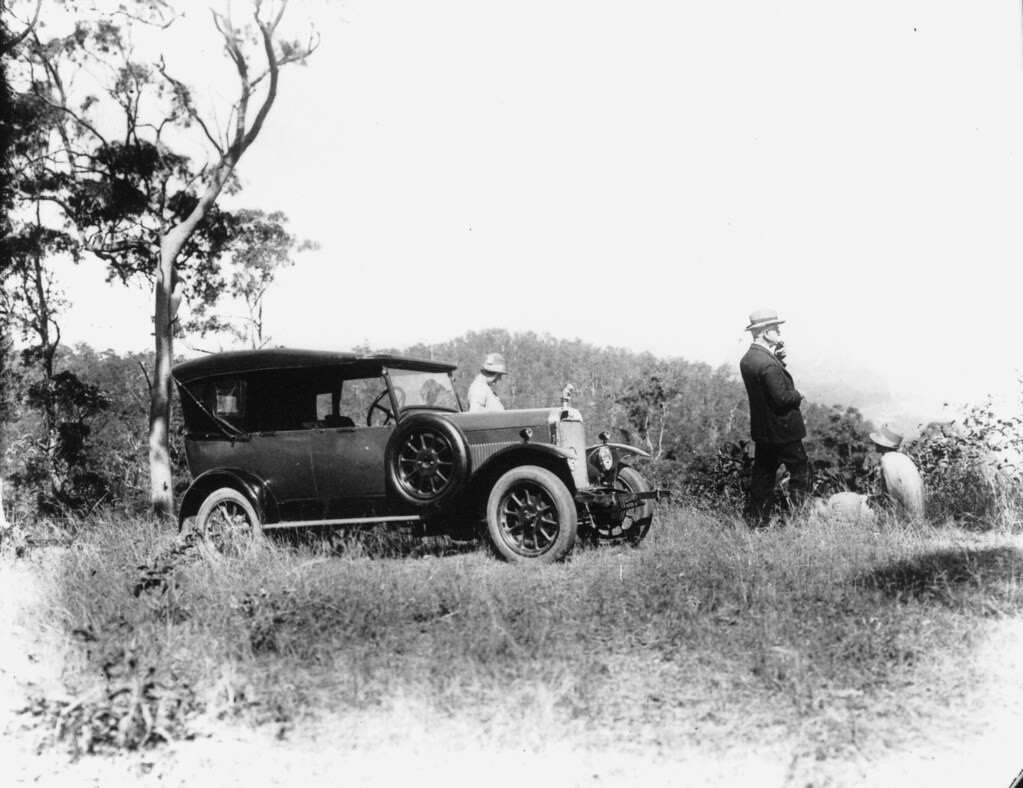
xmin=868 ymin=422 xmax=904 ymax=449
xmin=482 ymin=350 xmax=507 ymax=373
xmin=744 ymin=307 xmax=784 ymax=331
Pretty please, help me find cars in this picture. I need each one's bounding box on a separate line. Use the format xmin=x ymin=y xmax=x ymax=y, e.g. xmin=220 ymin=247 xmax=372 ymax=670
xmin=167 ymin=347 xmax=671 ymax=564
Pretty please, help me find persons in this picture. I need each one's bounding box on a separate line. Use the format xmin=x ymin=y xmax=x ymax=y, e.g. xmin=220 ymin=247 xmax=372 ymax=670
xmin=467 ymin=353 xmax=508 ymax=412
xmin=869 ymin=422 xmax=925 ymax=520
xmin=740 ymin=307 xmax=816 ymax=533
